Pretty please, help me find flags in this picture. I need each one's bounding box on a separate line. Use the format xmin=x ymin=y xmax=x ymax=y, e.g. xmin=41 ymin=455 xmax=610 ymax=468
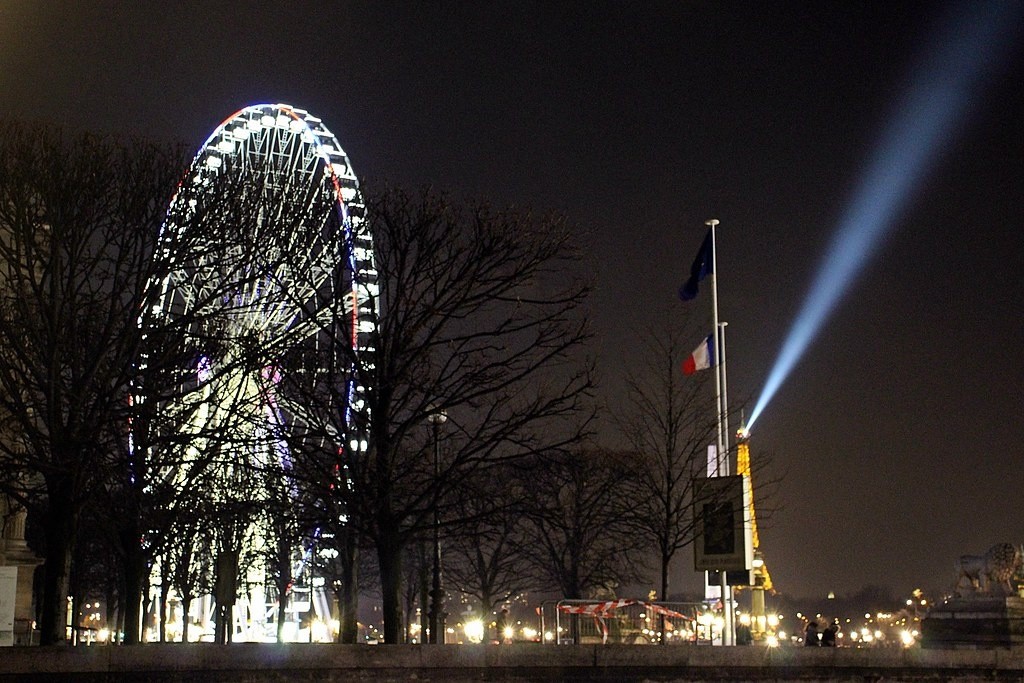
xmin=682 ymin=327 xmax=722 ymax=376
xmin=677 ymin=226 xmax=714 ymax=301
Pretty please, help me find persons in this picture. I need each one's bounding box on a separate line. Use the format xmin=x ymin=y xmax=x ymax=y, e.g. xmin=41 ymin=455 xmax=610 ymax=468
xmin=495 ymin=608 xmax=510 ymax=644
xmin=737 ymin=622 xmax=841 ymax=648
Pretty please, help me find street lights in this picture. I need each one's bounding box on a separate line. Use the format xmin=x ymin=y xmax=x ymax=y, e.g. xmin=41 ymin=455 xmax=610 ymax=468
xmin=422 ymin=400 xmax=451 ymax=646
